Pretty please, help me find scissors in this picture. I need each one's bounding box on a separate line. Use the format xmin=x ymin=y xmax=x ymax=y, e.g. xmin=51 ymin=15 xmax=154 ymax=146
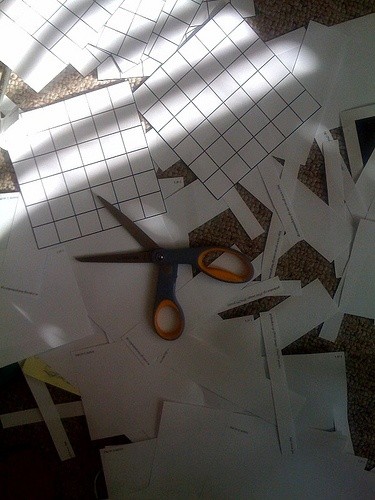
xmin=74 ymin=196 xmax=254 ymax=340
